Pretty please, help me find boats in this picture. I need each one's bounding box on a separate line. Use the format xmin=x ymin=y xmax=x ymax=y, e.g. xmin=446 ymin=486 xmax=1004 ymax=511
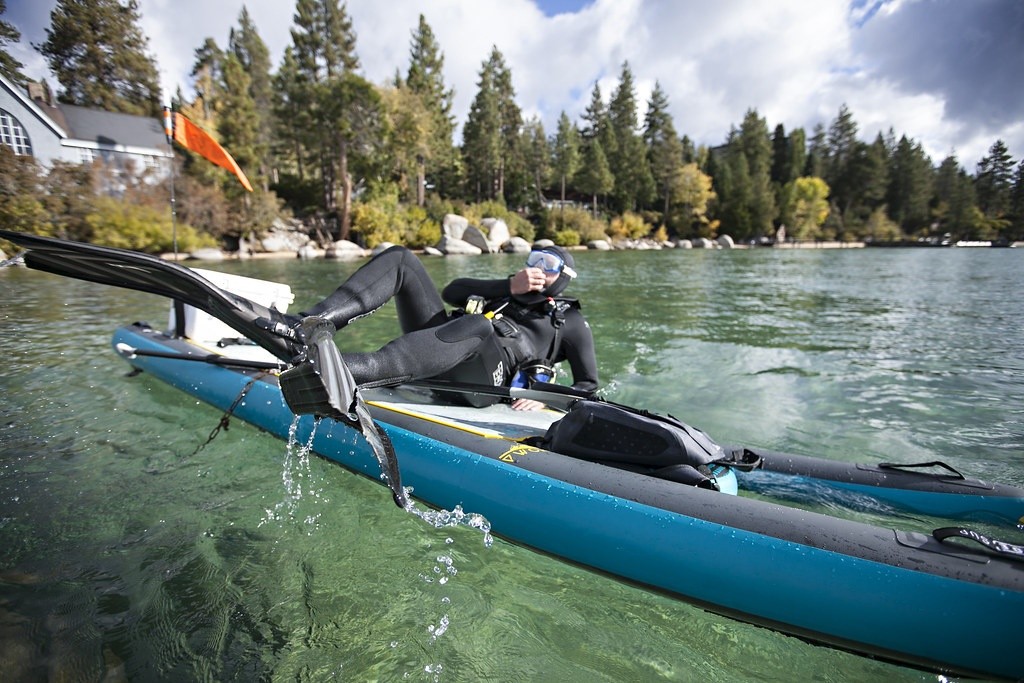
xmin=112 ymin=101 xmax=1024 ymax=683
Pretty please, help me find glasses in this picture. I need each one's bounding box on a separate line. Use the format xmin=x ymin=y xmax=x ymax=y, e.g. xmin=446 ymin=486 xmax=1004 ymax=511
xmin=526 ymin=250 xmax=564 ymax=274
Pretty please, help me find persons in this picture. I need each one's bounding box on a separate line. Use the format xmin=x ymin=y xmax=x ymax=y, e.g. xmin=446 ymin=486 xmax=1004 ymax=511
xmin=0 ymin=229 xmax=599 ymax=508
